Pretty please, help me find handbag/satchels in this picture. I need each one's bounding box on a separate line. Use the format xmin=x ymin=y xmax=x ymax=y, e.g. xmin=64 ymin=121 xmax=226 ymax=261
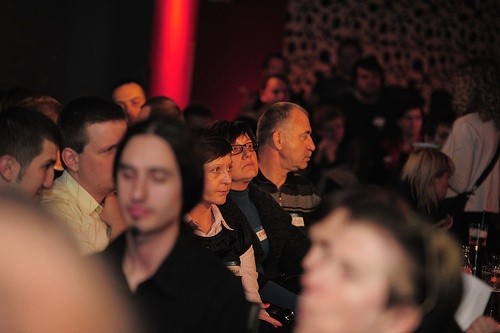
xmin=426 ymin=189 xmax=476 ymax=225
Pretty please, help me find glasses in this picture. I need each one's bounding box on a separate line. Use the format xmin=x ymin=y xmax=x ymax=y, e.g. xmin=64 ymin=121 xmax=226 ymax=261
xmin=229 ymin=142 xmax=260 ymax=155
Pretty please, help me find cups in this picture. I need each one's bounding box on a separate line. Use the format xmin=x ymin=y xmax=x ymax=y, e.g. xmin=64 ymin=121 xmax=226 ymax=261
xmin=469 ymin=223 xmax=488 ymax=270
xmin=481 ymin=265 xmax=500 ymax=321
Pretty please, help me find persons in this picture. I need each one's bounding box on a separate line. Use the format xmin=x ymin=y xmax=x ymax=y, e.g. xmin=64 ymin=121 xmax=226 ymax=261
xmin=84 ymin=117 xmax=247 ymax=333
xmin=0 ymin=193 xmax=145 ymax=332
xmin=290 ymin=188 xmax=499 ymax=333
xmin=37 ymin=98 xmax=132 ymax=257
xmin=182 ymin=128 xmax=282 ymax=333
xmin=211 ymin=119 xmax=312 ymax=333
xmin=15 ymin=55 xmax=499 ymax=231
xmin=0 ymin=109 xmax=61 ymax=205
xmin=253 ymin=102 xmax=345 ymax=255
